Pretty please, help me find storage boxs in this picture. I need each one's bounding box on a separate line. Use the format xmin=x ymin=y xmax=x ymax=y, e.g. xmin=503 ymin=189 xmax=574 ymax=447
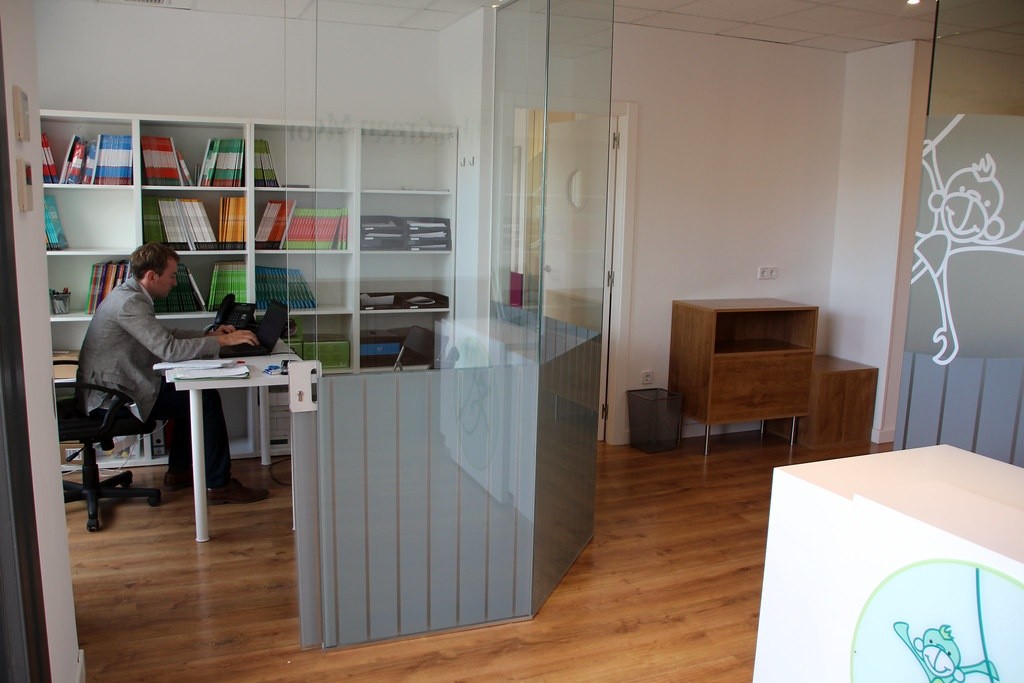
xmin=58 ymin=440 xmax=83 ymax=465
xmin=255 ymin=314 xmax=434 ymax=367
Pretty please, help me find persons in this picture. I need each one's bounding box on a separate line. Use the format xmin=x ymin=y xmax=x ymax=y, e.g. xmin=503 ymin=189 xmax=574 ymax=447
xmin=74 ymin=242 xmax=269 ymax=506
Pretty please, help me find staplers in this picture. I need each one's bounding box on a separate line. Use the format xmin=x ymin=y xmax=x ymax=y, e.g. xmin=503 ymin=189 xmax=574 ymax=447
xmin=281 ymin=359 xmax=299 ymax=374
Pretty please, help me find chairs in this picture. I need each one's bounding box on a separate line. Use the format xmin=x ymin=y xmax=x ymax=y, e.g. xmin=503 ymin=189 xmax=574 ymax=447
xmin=51 ymin=358 xmax=162 ymax=532
xmin=393 ymin=324 xmax=449 ymax=371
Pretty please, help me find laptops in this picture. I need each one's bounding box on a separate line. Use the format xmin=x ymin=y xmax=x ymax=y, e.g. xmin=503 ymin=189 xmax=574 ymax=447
xmin=219 ymin=299 xmax=291 ymax=358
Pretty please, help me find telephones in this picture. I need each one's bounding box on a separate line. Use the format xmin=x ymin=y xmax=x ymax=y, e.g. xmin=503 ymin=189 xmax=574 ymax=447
xmin=215 ymin=293 xmax=256 ymax=328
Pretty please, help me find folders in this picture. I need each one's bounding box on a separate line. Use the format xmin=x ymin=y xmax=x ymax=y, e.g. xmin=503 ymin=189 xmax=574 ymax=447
xmin=365 ymin=212 xmax=451 ymax=252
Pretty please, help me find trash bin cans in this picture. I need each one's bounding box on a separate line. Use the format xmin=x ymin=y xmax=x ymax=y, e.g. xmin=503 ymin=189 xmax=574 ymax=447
xmin=626 ymin=387 xmax=685 ymax=453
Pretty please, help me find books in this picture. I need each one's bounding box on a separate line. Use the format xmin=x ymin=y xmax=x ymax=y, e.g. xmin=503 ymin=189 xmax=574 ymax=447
xmin=41 ymin=130 xmax=348 ymax=315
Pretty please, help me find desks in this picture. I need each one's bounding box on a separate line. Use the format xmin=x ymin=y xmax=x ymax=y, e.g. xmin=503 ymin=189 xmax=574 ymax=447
xmin=750 ymin=441 xmax=1023 ymax=682
xmin=168 ymin=337 xmax=305 ymax=541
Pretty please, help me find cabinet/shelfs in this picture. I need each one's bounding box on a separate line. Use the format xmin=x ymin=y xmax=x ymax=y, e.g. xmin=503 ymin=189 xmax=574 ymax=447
xmin=763 ymin=352 xmax=880 ymax=450
xmin=38 ymin=109 xmax=460 ymax=473
xmin=669 ymin=297 xmax=821 ymax=460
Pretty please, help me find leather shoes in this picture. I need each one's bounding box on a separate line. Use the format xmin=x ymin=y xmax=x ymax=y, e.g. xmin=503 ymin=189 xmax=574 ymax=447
xmin=164 ymin=467 xmax=193 ymax=491
xmin=207 ymin=480 xmax=269 ymax=506
